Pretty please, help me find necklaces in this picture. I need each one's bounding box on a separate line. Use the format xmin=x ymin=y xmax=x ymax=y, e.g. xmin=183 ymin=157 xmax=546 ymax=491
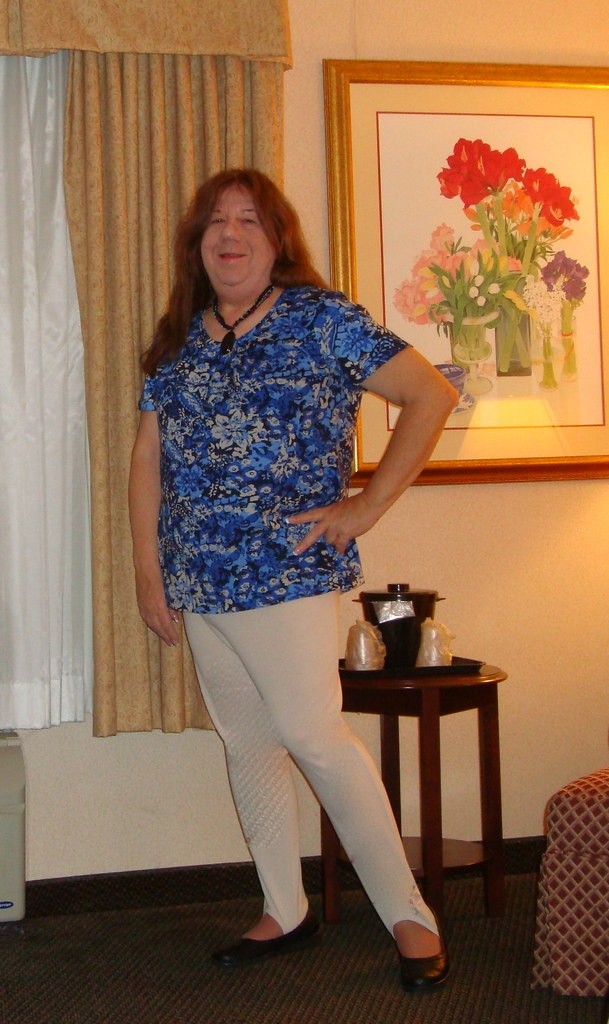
xmin=212 ymin=283 xmax=277 ymax=354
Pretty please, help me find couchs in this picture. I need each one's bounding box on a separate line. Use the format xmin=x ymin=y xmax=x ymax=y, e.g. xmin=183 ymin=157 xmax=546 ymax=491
xmin=529 ymin=766 xmax=609 ymax=998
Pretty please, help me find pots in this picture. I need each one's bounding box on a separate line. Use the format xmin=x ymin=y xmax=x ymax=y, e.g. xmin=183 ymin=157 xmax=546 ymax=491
xmin=352 ymin=584 xmax=446 ymax=666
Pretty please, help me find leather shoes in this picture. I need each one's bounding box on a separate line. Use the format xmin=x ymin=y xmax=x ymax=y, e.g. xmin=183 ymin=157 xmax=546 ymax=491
xmin=394 ymin=906 xmax=452 ymax=991
xmin=211 ymin=904 xmax=320 ymax=967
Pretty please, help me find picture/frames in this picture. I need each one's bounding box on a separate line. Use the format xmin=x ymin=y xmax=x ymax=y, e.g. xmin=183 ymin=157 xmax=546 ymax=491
xmin=322 ymin=56 xmax=609 ymax=489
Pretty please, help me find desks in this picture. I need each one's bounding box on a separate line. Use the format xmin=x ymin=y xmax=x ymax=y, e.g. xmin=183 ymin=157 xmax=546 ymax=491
xmin=320 ymin=665 xmax=509 ymax=925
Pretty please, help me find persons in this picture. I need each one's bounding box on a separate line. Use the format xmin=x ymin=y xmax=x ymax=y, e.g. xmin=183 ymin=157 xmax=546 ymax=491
xmin=128 ymin=167 xmax=460 ymax=990
